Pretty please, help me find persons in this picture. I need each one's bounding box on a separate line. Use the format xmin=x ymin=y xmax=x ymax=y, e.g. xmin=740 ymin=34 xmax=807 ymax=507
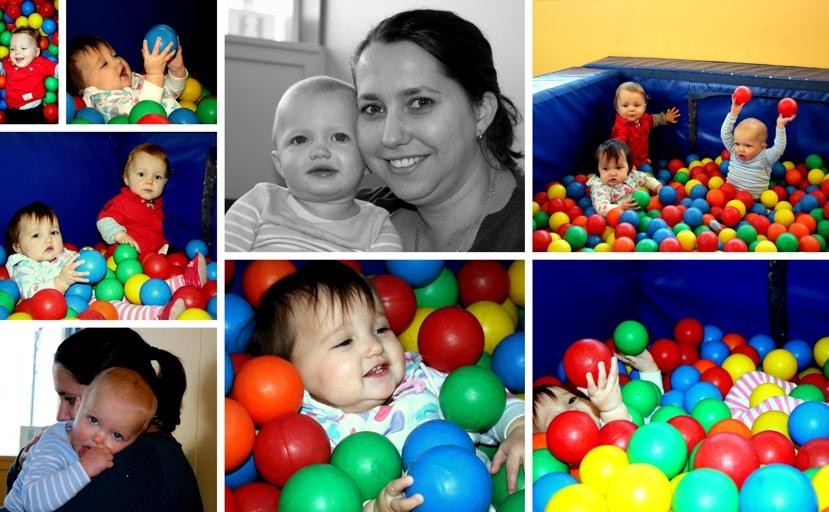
xmin=354 ymin=10 xmax=525 ymax=252
xmin=6 ymin=328 xmax=204 ymax=511
xmin=777 ymin=97 xmax=798 ymax=117
xmin=0 ymin=26 xmax=58 ymax=124
xmin=0 ymin=366 xmax=158 ymax=512
xmin=42 ymin=76 xmax=58 ymax=124
xmin=0 ymin=75 xmax=8 ymax=123
xmin=733 ymin=85 xmax=752 ymax=105
xmin=66 ymin=71 xmax=218 ymax=124
xmin=612 ymin=80 xmax=681 ymax=170
xmin=94 ymin=141 xmax=173 ymax=255
xmin=532 ymin=317 xmax=829 ymax=512
xmin=0 ymin=0 xmax=58 ymax=61
xmin=586 ymin=138 xmax=665 ymax=219
xmin=4 ymin=200 xmax=208 ymax=320
xmin=144 ymin=23 xmax=179 ymax=56
xmin=533 ymin=149 xmax=829 ymax=252
xmin=0 ymin=238 xmax=217 ymax=320
xmin=224 ymin=260 xmax=525 ymax=511
xmin=66 ymin=34 xmax=189 ymax=124
xmin=224 ymin=75 xmax=405 ymax=252
xmin=719 ymin=94 xmax=797 ymax=203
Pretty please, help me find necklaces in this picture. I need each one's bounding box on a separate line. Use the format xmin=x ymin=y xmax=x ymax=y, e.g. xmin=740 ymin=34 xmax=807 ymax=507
xmin=413 ymin=157 xmax=499 ymax=252
